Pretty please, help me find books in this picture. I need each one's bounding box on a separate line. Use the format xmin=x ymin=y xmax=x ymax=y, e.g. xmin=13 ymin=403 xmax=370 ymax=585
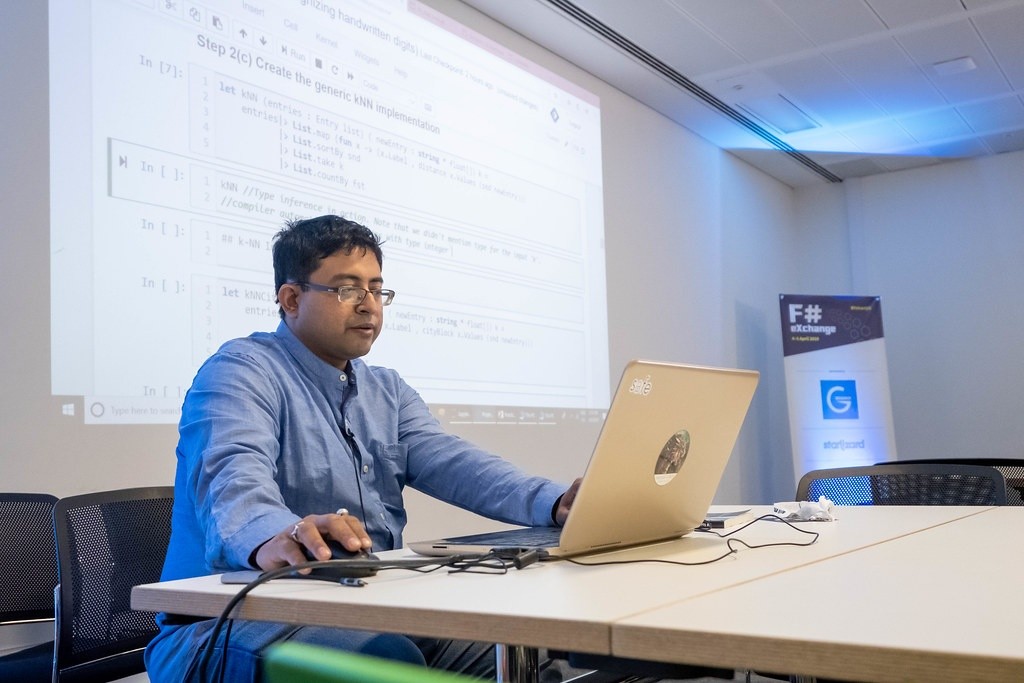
xmin=695 ymin=507 xmax=755 ymax=529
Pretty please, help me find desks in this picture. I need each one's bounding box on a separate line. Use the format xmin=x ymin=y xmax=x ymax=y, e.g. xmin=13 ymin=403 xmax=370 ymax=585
xmin=611 ymin=506 xmax=1024 ymax=683
xmin=131 ymin=504 xmax=999 ymax=683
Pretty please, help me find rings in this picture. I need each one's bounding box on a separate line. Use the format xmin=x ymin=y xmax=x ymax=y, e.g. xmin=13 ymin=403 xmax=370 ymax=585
xmin=336 ymin=508 xmax=348 ymax=517
xmin=291 ymin=521 xmax=304 ymax=541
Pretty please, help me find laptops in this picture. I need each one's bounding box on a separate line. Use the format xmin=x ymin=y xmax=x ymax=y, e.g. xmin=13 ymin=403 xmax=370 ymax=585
xmin=407 ymin=361 xmax=760 ymax=561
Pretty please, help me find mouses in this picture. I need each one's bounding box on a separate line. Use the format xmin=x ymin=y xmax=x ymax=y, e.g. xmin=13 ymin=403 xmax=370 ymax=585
xmin=281 ymin=535 xmax=380 ymax=579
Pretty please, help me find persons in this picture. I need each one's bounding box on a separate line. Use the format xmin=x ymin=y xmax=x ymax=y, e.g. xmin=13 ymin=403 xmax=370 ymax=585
xmin=147 ymin=214 xmax=583 ymax=683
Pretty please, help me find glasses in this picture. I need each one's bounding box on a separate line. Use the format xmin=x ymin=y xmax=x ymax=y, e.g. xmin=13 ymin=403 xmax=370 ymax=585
xmin=289 ymin=281 xmax=395 ymax=306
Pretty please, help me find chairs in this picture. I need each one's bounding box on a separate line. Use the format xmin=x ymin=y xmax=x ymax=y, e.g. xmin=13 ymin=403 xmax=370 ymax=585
xmin=795 ymin=465 xmax=1007 ymax=506
xmin=0 ymin=493 xmax=60 ymax=683
xmin=871 ymin=458 xmax=1024 ymax=506
xmin=52 ymin=486 xmax=175 ymax=683
